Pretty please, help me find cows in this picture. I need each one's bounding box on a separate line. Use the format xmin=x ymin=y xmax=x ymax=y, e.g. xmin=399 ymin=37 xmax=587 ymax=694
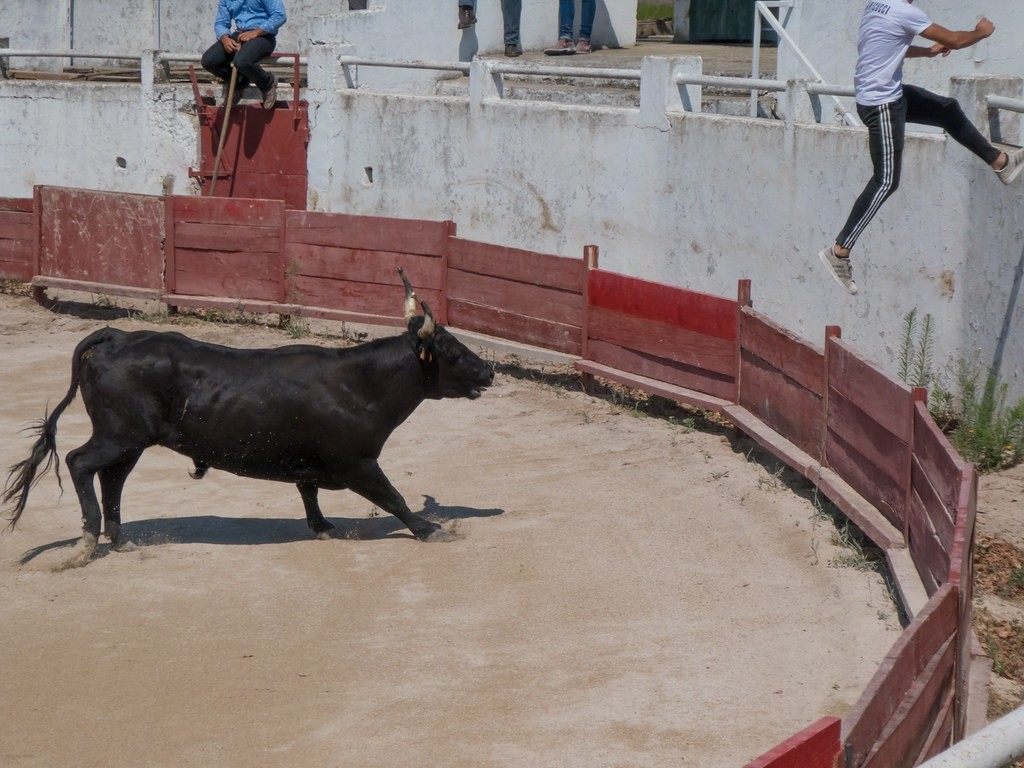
xmin=0 ymin=264 xmax=496 ymax=555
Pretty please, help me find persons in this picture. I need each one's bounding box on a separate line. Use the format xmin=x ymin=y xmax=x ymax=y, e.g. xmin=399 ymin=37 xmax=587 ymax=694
xmin=543 ymin=0 xmax=596 ymax=55
xmin=458 ymin=0 xmax=522 ymax=57
xmin=201 ymin=0 xmax=287 ymax=109
xmin=818 ymin=0 xmax=1024 ymax=296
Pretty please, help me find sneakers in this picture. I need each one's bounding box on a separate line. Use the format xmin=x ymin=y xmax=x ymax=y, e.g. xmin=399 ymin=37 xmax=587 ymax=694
xmin=819 ymin=246 xmax=857 ymax=295
xmin=994 ymin=147 xmax=1024 ymax=184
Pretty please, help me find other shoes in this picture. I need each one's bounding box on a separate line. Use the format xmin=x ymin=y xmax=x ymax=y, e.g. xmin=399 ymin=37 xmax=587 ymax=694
xmin=262 ymin=72 xmax=278 ymax=112
xmin=224 ymin=80 xmax=249 ymax=108
xmin=576 ymin=41 xmax=591 ymax=54
xmin=544 ymin=38 xmax=576 ymax=55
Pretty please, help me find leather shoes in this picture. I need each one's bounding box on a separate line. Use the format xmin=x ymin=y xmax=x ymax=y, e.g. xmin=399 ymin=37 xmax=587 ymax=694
xmin=457 ymin=6 xmax=477 ymax=29
xmin=505 ymin=44 xmax=518 ymax=57
